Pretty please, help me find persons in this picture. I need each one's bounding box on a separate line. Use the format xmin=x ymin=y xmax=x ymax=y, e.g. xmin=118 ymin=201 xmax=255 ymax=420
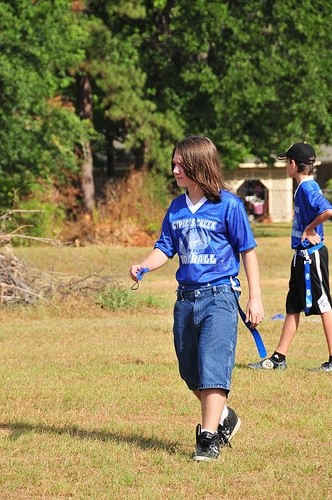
xmin=246 ymin=142 xmax=332 ymax=374
xmin=130 ymin=137 xmax=265 ymax=462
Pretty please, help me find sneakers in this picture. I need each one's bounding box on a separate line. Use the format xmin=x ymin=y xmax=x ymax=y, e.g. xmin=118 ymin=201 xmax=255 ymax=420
xmin=217 ymin=404 xmax=240 ymax=449
xmin=313 ymin=361 xmax=332 ymax=372
xmin=248 ymin=357 xmax=286 ymax=371
xmin=194 ymin=423 xmax=220 ymax=462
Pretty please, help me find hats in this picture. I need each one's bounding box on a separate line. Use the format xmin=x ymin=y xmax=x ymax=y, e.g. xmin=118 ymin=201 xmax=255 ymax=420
xmin=276 ymin=142 xmax=316 ymax=164
